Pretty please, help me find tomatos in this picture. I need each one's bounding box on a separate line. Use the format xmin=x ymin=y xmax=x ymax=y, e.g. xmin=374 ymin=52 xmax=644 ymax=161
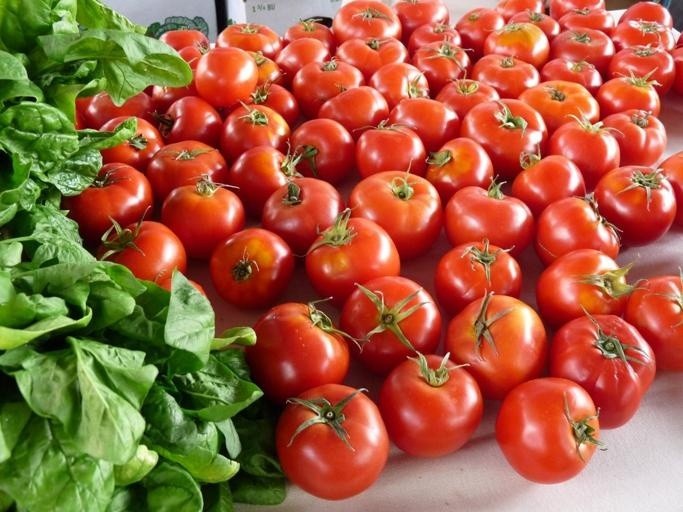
xmin=62 ymin=1 xmax=683 ymax=500
